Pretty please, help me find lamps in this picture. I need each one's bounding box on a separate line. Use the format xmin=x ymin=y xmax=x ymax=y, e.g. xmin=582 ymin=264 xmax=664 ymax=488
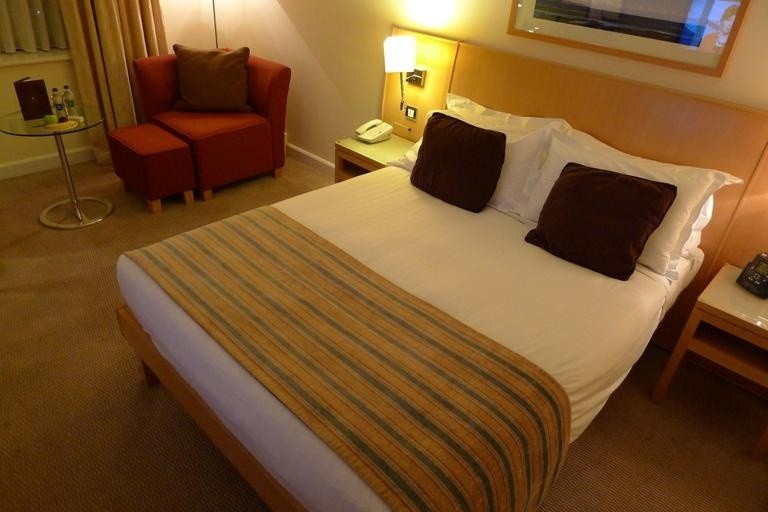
xmin=384 ymin=36 xmax=419 ymax=121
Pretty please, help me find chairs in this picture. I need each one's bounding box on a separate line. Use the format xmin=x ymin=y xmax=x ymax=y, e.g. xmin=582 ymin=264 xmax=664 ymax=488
xmin=133 ymin=48 xmax=293 ymax=212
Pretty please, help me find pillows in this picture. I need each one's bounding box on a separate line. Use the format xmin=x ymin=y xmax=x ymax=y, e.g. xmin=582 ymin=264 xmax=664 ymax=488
xmin=447 ymin=93 xmax=570 ymax=167
xmin=173 ymin=43 xmax=256 ymax=114
xmin=685 ymin=195 xmax=713 ymax=258
xmin=409 ymin=112 xmax=506 ymax=213
xmin=522 ymin=161 xmax=677 ymax=281
xmin=385 ymin=110 xmax=547 ymax=218
xmin=520 ymin=124 xmax=745 ymax=281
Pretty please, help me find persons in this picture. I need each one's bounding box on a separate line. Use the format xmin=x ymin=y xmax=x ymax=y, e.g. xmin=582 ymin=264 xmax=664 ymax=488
xmin=699 ymin=5 xmax=739 ymax=55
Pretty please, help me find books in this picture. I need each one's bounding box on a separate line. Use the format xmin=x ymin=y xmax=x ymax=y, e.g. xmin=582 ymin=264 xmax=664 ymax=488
xmin=13 ymin=77 xmax=53 ymax=121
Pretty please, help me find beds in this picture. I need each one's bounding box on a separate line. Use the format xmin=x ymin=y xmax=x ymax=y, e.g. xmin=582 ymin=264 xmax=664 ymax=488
xmin=115 ymin=165 xmax=705 ymax=512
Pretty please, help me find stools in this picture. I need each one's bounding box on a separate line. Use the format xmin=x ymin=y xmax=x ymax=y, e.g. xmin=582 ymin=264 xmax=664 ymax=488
xmin=106 ymin=123 xmax=199 ymax=213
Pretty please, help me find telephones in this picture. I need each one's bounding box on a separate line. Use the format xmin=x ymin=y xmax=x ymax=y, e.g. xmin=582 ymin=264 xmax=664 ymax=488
xmin=354 ymin=118 xmax=394 ymax=144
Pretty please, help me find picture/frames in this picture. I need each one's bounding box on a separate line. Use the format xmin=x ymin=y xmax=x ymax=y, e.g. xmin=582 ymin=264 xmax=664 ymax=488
xmin=506 ymin=0 xmax=751 ymax=78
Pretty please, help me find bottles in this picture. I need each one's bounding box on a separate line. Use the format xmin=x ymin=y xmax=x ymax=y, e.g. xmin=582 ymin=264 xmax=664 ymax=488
xmin=51 ymin=85 xmax=75 ymax=122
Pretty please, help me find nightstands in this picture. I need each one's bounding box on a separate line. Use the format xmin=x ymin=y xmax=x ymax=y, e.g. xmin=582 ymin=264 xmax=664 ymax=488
xmin=651 ymin=263 xmax=768 ymax=460
xmin=335 ymin=132 xmax=415 ymax=184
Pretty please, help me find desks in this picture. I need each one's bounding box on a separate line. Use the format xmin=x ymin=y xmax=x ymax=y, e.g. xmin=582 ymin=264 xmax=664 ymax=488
xmin=0 ymin=105 xmax=113 ymax=229
